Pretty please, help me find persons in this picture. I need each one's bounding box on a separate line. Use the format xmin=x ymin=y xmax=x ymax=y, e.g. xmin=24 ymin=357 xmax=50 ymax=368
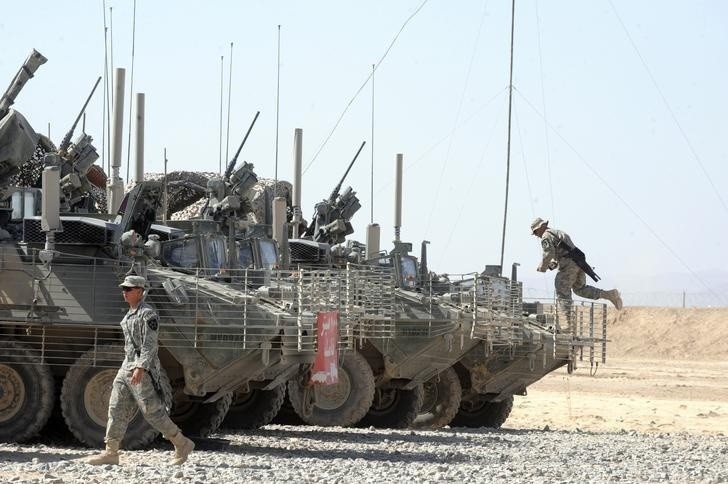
xmin=88 ymin=275 xmax=195 ymax=465
xmin=530 ymin=217 xmax=622 ymax=335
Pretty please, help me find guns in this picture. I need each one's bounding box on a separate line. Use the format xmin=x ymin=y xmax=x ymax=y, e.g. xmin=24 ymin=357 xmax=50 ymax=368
xmin=556 ymin=240 xmax=601 ymax=282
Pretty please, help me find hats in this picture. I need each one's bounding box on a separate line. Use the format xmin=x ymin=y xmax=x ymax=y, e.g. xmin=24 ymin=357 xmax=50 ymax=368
xmin=119 ymin=276 xmax=145 ymax=288
xmin=530 ymin=217 xmax=549 ymax=235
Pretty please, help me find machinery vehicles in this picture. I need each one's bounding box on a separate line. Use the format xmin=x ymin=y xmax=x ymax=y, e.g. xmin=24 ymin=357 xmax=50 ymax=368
xmin=0 ymin=0 xmax=613 ymax=450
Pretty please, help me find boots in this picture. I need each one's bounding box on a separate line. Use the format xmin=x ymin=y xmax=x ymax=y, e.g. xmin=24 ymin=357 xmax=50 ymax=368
xmin=89 ymin=441 xmax=120 ymax=464
xmin=600 ymin=289 xmax=622 ymax=310
xmin=167 ymin=432 xmax=195 ymax=466
